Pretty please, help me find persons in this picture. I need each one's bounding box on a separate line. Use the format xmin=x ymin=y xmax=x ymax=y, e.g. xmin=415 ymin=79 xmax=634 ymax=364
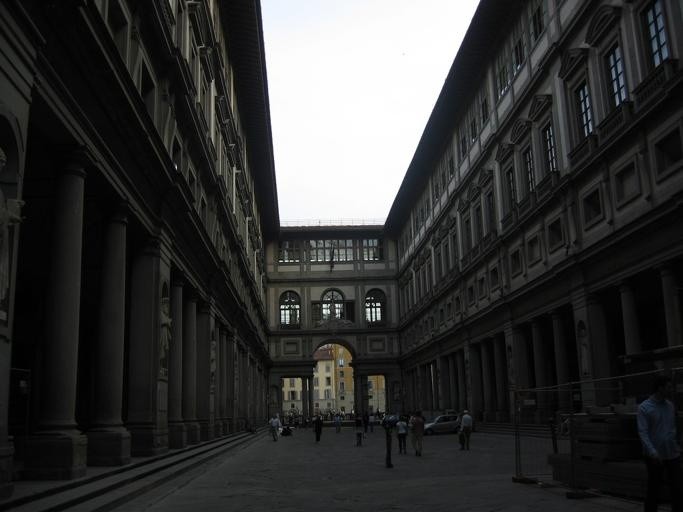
xmin=287 ymin=409 xmax=345 ymax=427
xmin=457 ymin=409 xmax=472 ymax=451
xmin=363 ymin=410 xmax=370 ymax=432
xmin=367 ymin=407 xmax=374 ymax=432
xmin=394 ymin=415 xmax=408 ymax=456
xmin=334 ymin=414 xmax=341 ymax=433
xmin=311 ymin=415 xmax=324 ymax=441
xmin=354 ymin=416 xmax=364 ymax=447
xmin=410 ymin=409 xmax=424 ymax=456
xmin=381 ymin=413 xmax=398 ymax=468
xmin=268 ymin=412 xmax=281 ymax=441
xmin=375 ymin=408 xmax=379 ymax=419
xmin=635 ymin=372 xmax=682 ymax=511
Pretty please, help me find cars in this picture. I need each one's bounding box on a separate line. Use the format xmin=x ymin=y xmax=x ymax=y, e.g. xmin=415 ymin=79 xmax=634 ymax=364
xmin=423 ymin=414 xmax=462 ymax=435
